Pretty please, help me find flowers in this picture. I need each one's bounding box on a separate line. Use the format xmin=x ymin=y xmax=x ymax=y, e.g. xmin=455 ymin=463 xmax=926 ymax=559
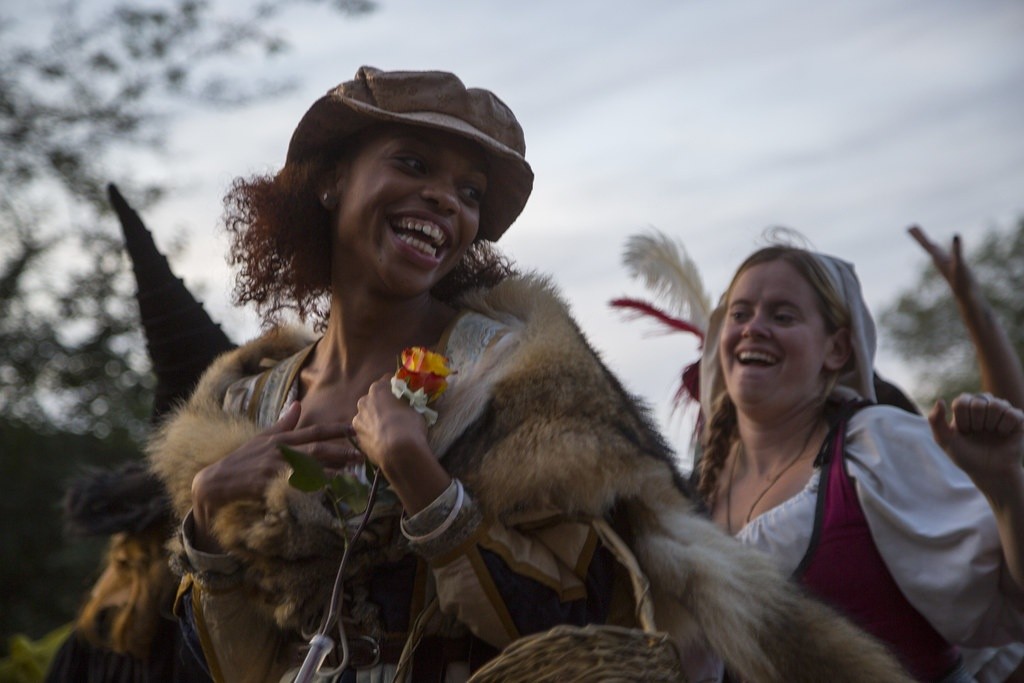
xmin=271 ymin=347 xmax=463 ymax=683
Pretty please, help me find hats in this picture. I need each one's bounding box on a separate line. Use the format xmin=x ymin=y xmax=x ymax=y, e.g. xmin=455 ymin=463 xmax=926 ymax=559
xmin=285 ymin=65 xmax=534 ymax=249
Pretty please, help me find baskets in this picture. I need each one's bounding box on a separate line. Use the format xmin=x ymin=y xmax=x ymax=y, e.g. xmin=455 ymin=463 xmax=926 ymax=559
xmin=372 ymin=512 xmax=692 ymax=683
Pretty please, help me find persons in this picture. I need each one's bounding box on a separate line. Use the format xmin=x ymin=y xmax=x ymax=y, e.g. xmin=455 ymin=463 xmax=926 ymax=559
xmin=686 ymin=244 xmax=1024 ymax=683
xmin=902 ymin=227 xmax=1021 ymax=403
xmin=46 ymin=531 xmax=177 ymax=683
xmin=154 ymin=62 xmax=722 ymax=681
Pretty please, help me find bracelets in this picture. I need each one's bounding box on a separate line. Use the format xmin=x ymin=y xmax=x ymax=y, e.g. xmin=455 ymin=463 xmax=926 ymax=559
xmin=398 ymin=480 xmax=464 ymax=542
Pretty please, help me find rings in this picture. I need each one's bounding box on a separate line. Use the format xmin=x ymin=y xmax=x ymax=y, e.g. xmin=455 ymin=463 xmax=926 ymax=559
xmin=974 ymin=393 xmax=990 ymax=405
